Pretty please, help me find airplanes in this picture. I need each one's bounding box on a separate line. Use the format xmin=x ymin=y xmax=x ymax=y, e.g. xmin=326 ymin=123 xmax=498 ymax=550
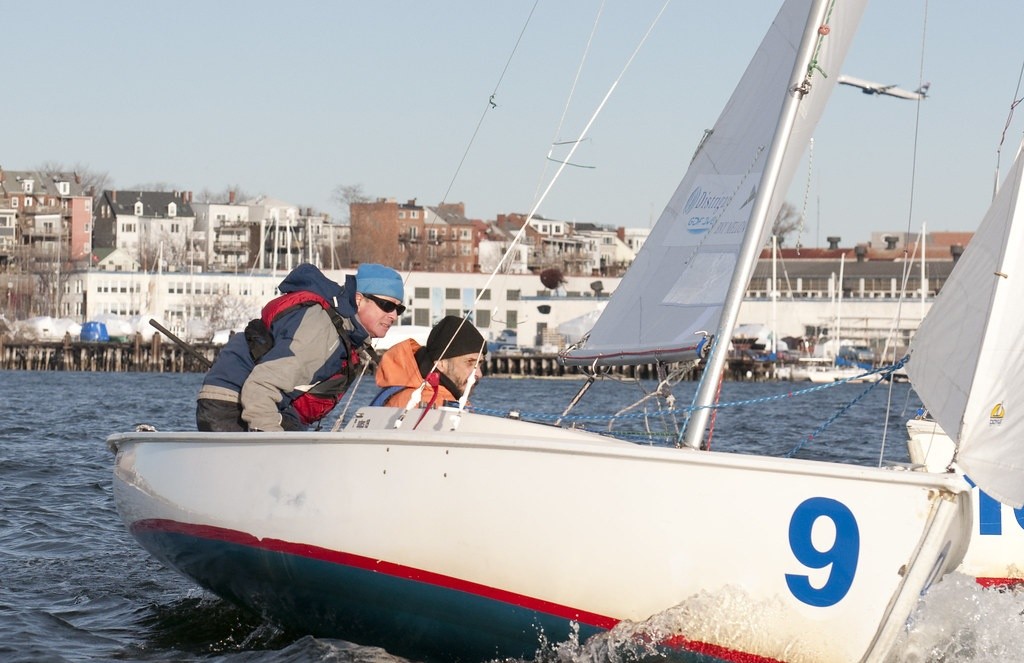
xmin=834 ymin=74 xmax=932 ymax=102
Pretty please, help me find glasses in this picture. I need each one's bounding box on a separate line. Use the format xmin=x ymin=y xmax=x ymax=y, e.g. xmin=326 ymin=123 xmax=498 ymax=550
xmin=363 ymin=294 xmax=406 ymax=316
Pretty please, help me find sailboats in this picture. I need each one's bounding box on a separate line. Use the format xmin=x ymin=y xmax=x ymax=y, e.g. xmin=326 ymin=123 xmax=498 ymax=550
xmin=105 ymin=0 xmax=1023 ymax=662
xmin=765 ymin=218 xmax=934 ymax=386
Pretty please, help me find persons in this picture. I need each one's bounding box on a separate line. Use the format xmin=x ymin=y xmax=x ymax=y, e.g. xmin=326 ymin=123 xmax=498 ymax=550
xmin=368 ymin=315 xmax=488 ymax=411
xmin=194 ymin=262 xmax=406 ymax=432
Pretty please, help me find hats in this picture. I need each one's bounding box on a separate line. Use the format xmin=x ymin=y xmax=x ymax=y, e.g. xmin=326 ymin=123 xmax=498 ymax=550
xmin=354 ymin=263 xmax=403 ymax=302
xmin=427 ymin=316 xmax=487 ymax=361
